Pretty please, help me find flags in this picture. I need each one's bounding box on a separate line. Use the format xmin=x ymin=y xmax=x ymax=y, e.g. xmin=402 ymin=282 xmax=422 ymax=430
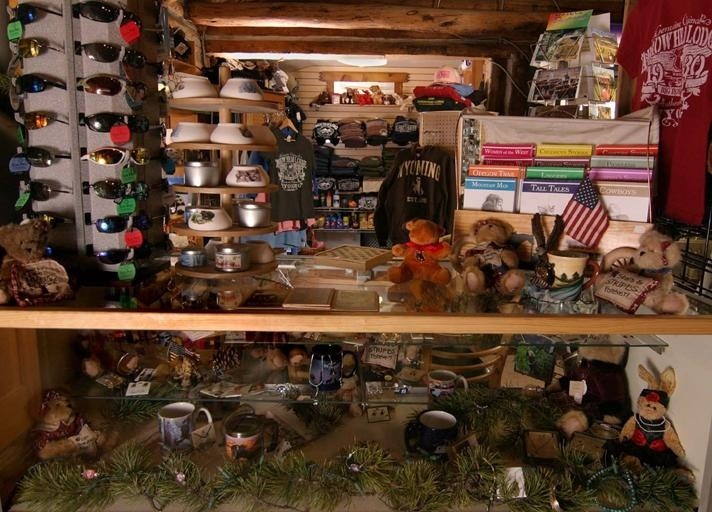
xmin=561 ymin=177 xmax=610 ymax=248
xmin=168 ymin=341 xmax=200 ymax=368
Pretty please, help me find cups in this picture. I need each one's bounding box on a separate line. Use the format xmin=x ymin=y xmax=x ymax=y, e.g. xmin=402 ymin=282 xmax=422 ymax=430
xmin=404 ymin=410 xmax=458 ymax=462
xmin=307 ymin=342 xmax=359 ymax=392
xmin=156 ymin=401 xmax=213 ymax=456
xmin=545 ymin=250 xmax=600 ymax=301
xmin=428 ymin=370 xmax=469 ymax=405
xmin=222 ymin=411 xmax=279 ymax=467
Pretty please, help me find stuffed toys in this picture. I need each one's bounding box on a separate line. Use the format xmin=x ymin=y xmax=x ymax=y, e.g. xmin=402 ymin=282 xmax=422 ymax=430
xmin=618 ymin=363 xmax=685 ymax=468
xmin=544 ymin=333 xmax=633 ymax=436
xmin=463 ymin=218 xmax=525 ymax=297
xmin=389 ymin=219 xmax=453 ymax=285
xmin=594 ymin=229 xmax=689 ymax=315
xmin=27 ymin=387 xmax=107 ymax=466
xmin=1 ymin=218 xmax=73 ymax=306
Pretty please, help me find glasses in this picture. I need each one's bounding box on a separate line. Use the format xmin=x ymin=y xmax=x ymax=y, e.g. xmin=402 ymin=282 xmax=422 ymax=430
xmin=7 ymin=1 xmax=173 ymax=265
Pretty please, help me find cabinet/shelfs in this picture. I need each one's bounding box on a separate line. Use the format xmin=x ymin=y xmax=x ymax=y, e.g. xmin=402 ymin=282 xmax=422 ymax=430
xmin=2 ymin=3 xmax=171 ymax=276
xmin=167 ymin=64 xmax=287 ymax=289
xmin=454 ymin=111 xmax=659 ymax=263
xmin=2 ymin=262 xmax=712 ymax=512
xmin=288 ymin=56 xmax=491 ymax=258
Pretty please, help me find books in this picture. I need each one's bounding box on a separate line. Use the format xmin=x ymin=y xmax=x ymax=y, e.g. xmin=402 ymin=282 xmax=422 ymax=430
xmin=463 ymin=143 xmax=658 ymax=222
xmin=526 ymin=27 xmax=619 ymax=121
xmin=236 ymin=286 xmax=380 ymax=312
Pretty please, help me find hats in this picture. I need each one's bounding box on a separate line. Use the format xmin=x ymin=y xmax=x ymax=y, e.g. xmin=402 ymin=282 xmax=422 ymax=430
xmin=431 ymin=69 xmax=463 ymax=87
